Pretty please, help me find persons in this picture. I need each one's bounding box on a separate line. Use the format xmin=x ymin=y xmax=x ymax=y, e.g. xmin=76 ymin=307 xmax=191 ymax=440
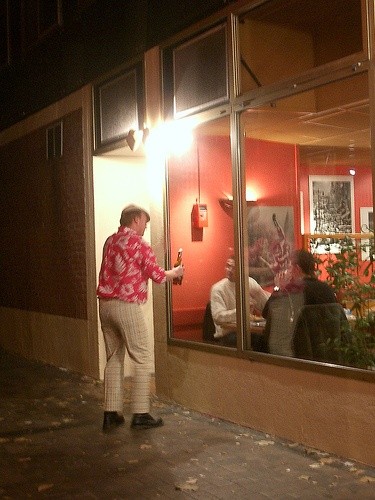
xmin=262 ymin=248 xmax=350 ymax=354
xmin=96 ymin=205 xmax=185 ymax=432
xmin=210 ymin=254 xmax=269 ymax=353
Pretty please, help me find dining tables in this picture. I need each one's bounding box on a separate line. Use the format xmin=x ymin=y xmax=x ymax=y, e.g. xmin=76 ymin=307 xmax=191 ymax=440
xmin=219 ymin=321 xmax=267 ymax=333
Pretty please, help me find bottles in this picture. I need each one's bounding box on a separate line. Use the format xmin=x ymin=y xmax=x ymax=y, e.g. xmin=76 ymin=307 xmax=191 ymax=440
xmin=172 ymin=248 xmax=183 ymax=285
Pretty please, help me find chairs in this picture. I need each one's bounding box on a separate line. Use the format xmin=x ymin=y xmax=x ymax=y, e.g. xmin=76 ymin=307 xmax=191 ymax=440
xmin=289 ymin=303 xmax=352 ymax=366
xmin=203 ymin=301 xmax=221 ymax=342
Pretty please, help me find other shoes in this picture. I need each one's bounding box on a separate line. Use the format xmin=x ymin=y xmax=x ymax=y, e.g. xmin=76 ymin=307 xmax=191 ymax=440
xmin=130 ymin=413 xmax=162 ymax=428
xmin=102 ymin=412 xmax=125 ymax=429
